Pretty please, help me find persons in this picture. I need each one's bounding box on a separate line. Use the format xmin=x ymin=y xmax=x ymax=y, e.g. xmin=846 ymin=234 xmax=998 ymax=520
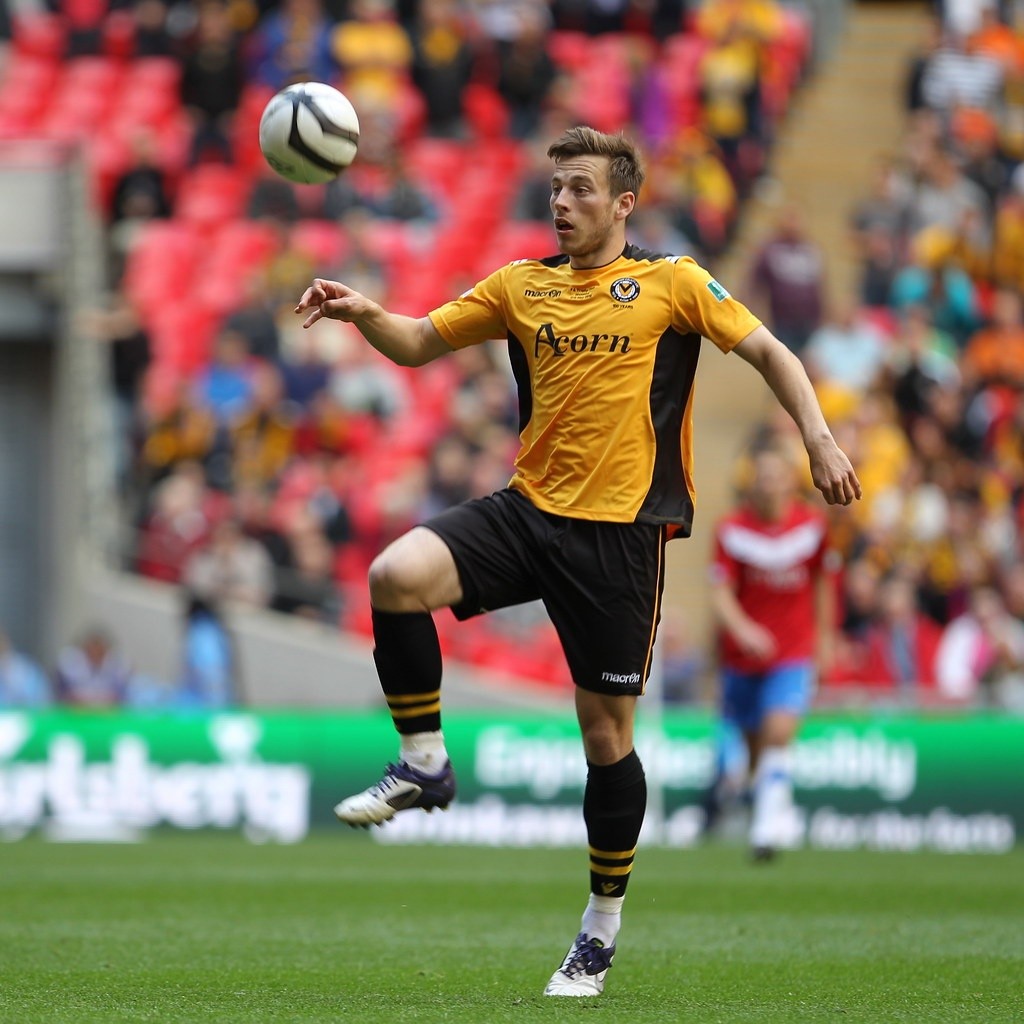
xmin=0 ymin=0 xmax=1022 ymax=719
xmin=293 ymin=124 xmax=865 ymax=997
xmin=697 ymin=426 xmax=847 ymax=867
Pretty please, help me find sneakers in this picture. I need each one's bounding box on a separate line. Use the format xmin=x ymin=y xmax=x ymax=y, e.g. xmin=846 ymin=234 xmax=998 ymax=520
xmin=542 ymin=932 xmax=616 ymax=998
xmin=333 ymin=756 xmax=456 ymax=829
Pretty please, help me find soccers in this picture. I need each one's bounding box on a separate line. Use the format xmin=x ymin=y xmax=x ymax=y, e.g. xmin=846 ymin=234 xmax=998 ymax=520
xmin=256 ymin=80 xmax=364 ymax=186
